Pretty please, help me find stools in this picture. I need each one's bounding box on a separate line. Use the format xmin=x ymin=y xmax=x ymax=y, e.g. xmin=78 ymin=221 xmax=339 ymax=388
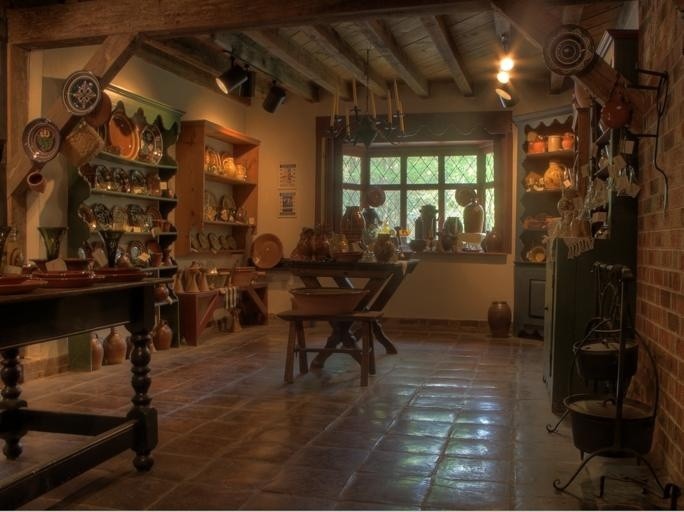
xmin=277 ymin=309 xmax=385 ymax=388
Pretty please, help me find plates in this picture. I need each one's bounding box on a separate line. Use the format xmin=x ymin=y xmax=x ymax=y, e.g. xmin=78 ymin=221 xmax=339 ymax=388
xmin=250 ymin=232 xmax=284 ymax=269
xmin=462 ymin=247 xmax=482 ymax=252
xmin=191 ymin=231 xmax=237 ymax=251
xmin=0 ymin=267 xmax=146 ymax=293
xmin=80 ymin=163 xmax=164 ymax=267
xmin=543 ymin=24 xmax=594 ymax=77
xmin=61 ymin=68 xmax=102 ymax=116
xmin=454 ymin=185 xmax=476 ymax=206
xmin=205 ymin=188 xmax=236 ymax=210
xmin=22 ymin=117 xmax=61 ymax=164
xmin=204 ymin=146 xmax=233 ymax=170
xmin=365 ymin=185 xmax=387 ymax=206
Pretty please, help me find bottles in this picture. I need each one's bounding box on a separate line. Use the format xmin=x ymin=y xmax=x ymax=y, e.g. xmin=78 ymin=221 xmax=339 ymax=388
xmin=302 ymin=231 xmax=322 ymax=263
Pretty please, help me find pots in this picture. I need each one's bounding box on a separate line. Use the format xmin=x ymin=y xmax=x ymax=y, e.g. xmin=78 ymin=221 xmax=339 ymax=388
xmin=564 ymin=335 xmax=654 ymax=457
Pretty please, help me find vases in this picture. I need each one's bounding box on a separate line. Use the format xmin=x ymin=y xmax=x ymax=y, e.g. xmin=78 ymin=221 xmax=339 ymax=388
xmin=342 ymin=204 xmax=366 ymax=242
xmin=488 ymin=300 xmax=512 ymax=336
xmin=407 ymin=197 xmax=501 ymax=253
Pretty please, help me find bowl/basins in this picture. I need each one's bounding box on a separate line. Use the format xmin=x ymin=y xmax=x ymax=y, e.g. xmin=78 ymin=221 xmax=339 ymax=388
xmin=108 ymin=109 xmax=164 ymax=165
xmin=334 ymin=252 xmax=362 ymax=264
xmin=288 ymin=287 xmax=370 ymax=314
xmin=205 ymin=266 xmax=256 ymax=288
xmin=410 ymin=239 xmax=430 ymax=253
xmin=27 ymin=256 xmax=92 ymax=273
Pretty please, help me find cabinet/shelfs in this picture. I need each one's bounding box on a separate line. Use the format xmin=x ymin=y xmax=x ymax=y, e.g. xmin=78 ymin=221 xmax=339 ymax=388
xmin=510 ymin=103 xmax=587 ymax=337
xmin=67 ymin=79 xmax=183 ymax=371
xmin=548 ymin=28 xmax=641 ymax=412
xmin=175 ymin=118 xmax=269 ymax=344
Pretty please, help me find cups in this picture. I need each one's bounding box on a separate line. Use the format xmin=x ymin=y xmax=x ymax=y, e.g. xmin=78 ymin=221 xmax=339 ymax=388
xmin=26 ymin=170 xmax=46 ymax=192
xmin=547 ymin=135 xmax=562 ymax=152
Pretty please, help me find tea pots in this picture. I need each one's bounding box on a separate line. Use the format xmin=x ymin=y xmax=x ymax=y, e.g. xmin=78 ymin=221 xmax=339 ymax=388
xmin=561 ymin=132 xmax=577 ymax=150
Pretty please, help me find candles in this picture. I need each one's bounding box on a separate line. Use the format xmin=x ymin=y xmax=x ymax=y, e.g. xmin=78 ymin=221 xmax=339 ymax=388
xmin=330 ymin=72 xmax=404 ymax=138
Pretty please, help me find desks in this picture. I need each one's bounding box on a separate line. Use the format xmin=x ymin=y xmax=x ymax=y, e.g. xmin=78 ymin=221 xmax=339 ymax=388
xmin=0 ymin=276 xmax=176 ymax=510
xmin=275 ymin=259 xmax=420 ymax=367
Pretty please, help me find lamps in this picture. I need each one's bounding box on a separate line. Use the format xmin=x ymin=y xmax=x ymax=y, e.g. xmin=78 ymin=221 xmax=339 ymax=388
xmin=239 ymin=60 xmax=259 ymax=98
xmin=262 ymin=82 xmax=287 ymax=113
xmin=496 ymin=81 xmax=519 ymax=109
xmin=215 ymin=50 xmax=247 ymax=94
